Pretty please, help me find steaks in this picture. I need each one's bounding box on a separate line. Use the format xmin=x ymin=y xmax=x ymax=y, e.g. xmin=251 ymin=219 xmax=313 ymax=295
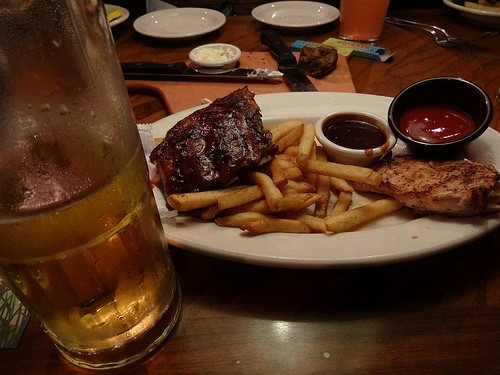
xmin=298 ymin=43 xmax=338 ymax=79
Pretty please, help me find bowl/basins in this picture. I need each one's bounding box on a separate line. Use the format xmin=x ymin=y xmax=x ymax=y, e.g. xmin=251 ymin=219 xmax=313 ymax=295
xmin=387 ymin=76 xmax=494 ymax=162
xmin=315 ymin=110 xmax=397 ymax=167
xmin=189 ymin=44 xmax=241 ymax=69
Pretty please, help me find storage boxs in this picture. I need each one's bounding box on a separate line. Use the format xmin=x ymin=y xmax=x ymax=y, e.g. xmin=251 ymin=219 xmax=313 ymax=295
xmin=291 ymin=37 xmax=397 ymax=63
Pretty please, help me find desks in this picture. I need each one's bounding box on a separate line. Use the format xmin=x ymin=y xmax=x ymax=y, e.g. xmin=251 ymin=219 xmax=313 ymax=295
xmin=0 ymin=8 xmax=500 ymax=375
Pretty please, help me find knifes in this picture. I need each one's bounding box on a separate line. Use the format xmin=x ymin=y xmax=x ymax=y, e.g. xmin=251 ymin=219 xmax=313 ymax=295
xmin=260 ymin=29 xmax=318 ymax=92
xmin=119 ymin=62 xmax=284 ymax=79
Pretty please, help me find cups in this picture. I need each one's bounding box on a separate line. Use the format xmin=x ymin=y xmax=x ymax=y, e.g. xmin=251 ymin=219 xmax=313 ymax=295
xmin=0 ymin=0 xmax=181 ymax=370
xmin=337 ymin=0 xmax=391 ymax=45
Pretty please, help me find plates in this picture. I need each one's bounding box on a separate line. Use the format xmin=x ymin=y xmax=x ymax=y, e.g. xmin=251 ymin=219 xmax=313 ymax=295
xmin=443 ymin=0 xmax=500 ymax=23
xmin=141 ymin=92 xmax=500 ymax=267
xmin=104 ymin=4 xmax=130 ymax=27
xmin=251 ymin=1 xmax=340 ymax=32
xmin=133 ymin=8 xmax=226 ymax=41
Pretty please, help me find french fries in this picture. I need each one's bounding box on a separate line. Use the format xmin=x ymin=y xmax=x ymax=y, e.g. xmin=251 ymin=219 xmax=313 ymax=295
xmin=151 ymin=118 xmax=402 ymax=235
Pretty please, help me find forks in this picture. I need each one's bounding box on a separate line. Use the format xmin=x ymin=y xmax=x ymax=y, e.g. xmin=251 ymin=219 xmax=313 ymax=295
xmin=384 ymin=17 xmax=499 ymax=48
